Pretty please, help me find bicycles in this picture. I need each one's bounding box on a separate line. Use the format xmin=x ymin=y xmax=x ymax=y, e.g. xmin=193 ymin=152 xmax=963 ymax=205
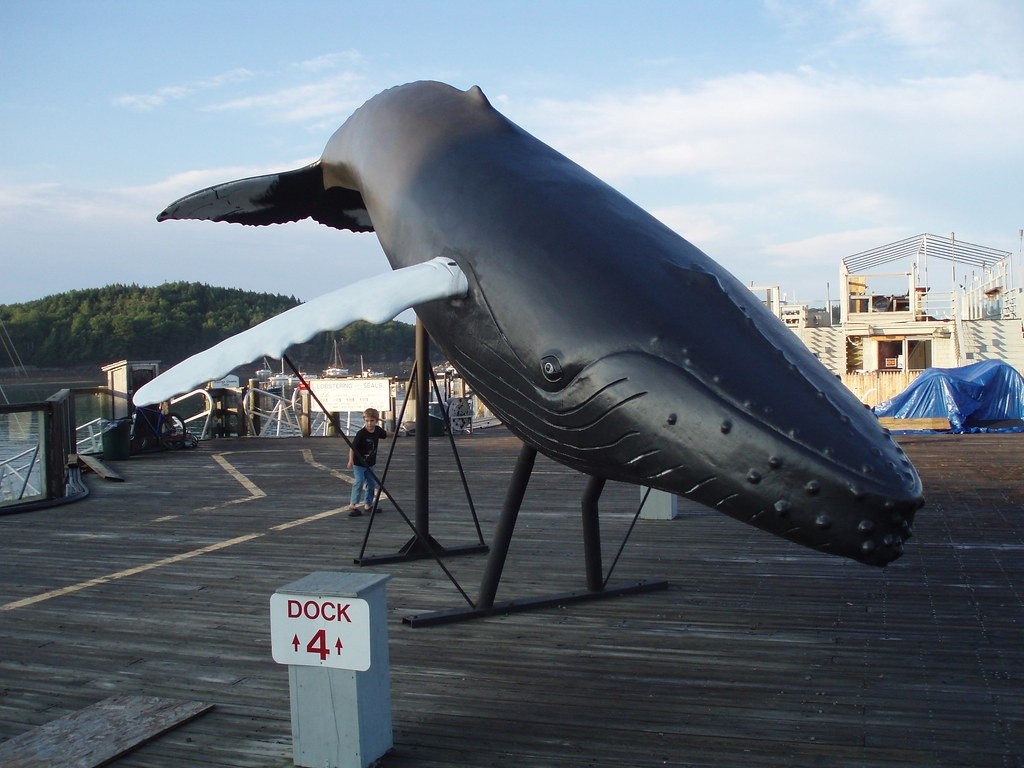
xmin=128 ymin=390 xmax=186 ymax=455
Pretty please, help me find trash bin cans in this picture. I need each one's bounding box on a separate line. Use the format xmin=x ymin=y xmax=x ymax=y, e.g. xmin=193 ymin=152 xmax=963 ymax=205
xmin=429 ymin=402 xmax=448 ymax=436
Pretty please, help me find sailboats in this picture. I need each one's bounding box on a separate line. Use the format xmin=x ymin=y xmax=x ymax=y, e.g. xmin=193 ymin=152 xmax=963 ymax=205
xmin=323 ymin=338 xmax=349 ymax=378
xmin=255 ymin=355 xmax=319 ymax=389
xmin=360 ymin=354 xmax=385 ymax=378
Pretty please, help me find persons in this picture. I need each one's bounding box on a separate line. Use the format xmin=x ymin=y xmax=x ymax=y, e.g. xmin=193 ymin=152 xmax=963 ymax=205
xmin=346 ymin=407 xmax=401 ymax=516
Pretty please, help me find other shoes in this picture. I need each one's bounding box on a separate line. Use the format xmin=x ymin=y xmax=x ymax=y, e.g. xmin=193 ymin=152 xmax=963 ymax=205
xmin=365 ymin=506 xmax=382 ymax=514
xmin=349 ymin=510 xmax=362 ymax=517
